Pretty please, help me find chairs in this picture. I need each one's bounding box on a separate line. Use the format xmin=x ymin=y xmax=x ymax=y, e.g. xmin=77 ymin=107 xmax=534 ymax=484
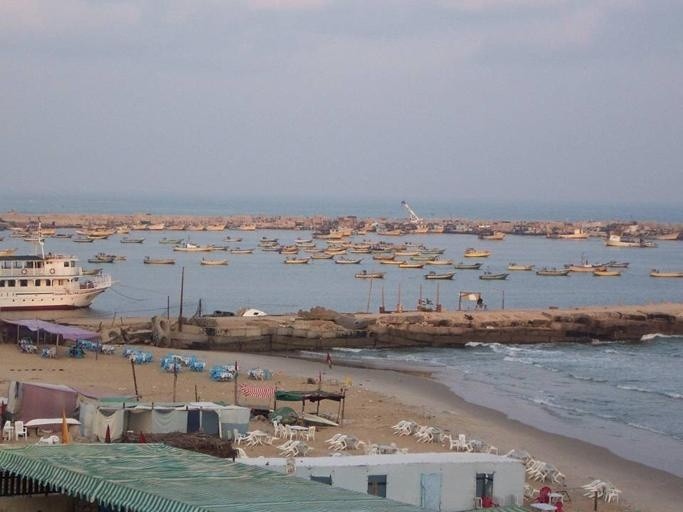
xmin=519 ymin=454 xmax=565 ymax=484
xmin=582 ymin=480 xmax=620 ymax=503
xmin=277 ymin=439 xmax=313 ymax=458
xmin=524 ymin=480 xmax=570 ymax=512
xmin=446 ymin=433 xmax=515 ymax=457
xmin=272 ymin=421 xmax=316 ymax=441
xmin=390 ymin=419 xmax=451 ymax=447
xmin=235 ymin=428 xmax=272 ymax=447
xmin=327 ymin=434 xmax=407 ymax=457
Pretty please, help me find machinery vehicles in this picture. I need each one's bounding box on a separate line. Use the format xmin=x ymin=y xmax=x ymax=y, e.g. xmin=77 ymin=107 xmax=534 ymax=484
xmin=401 ymin=197 xmax=426 ymax=228
